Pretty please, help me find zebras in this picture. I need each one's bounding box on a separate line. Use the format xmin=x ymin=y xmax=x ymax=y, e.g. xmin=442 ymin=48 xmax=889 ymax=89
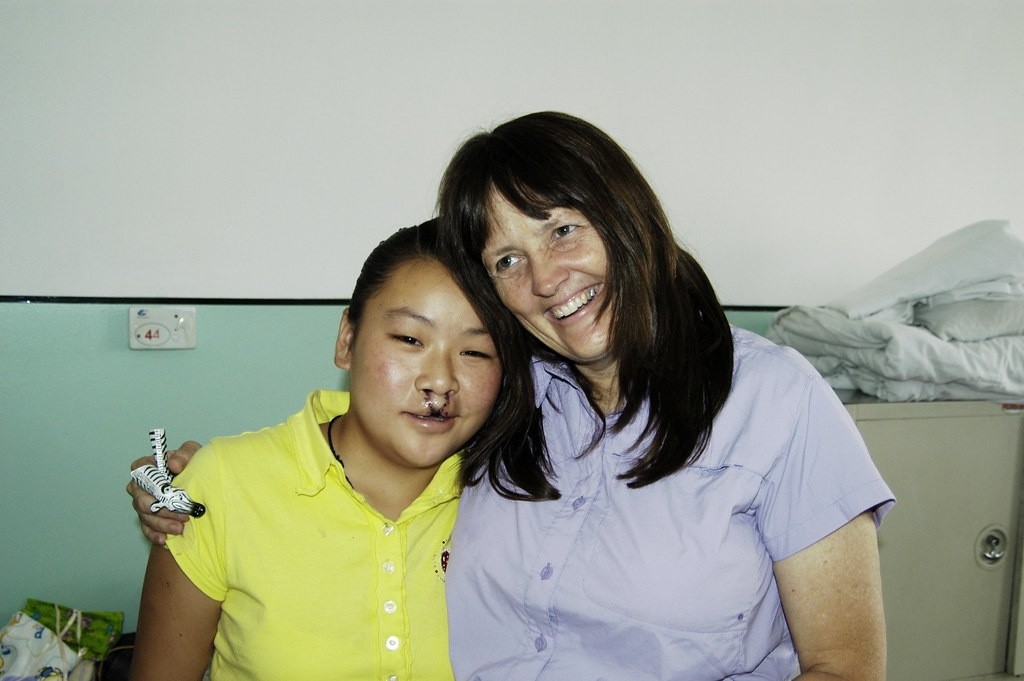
xmin=130 ymin=428 xmax=207 ymax=519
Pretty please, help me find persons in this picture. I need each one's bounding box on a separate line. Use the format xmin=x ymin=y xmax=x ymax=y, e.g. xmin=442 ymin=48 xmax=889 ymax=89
xmin=129 ymin=215 xmax=500 ymax=681
xmin=127 ymin=111 xmax=897 ymax=681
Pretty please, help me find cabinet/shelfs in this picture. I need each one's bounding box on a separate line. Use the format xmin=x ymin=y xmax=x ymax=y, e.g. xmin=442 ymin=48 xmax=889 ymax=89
xmin=839 ymin=392 xmax=1024 ymax=681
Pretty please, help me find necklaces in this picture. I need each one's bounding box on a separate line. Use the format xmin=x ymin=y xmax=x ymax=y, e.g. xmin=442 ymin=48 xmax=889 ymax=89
xmin=327 ymin=413 xmax=355 ymax=489
